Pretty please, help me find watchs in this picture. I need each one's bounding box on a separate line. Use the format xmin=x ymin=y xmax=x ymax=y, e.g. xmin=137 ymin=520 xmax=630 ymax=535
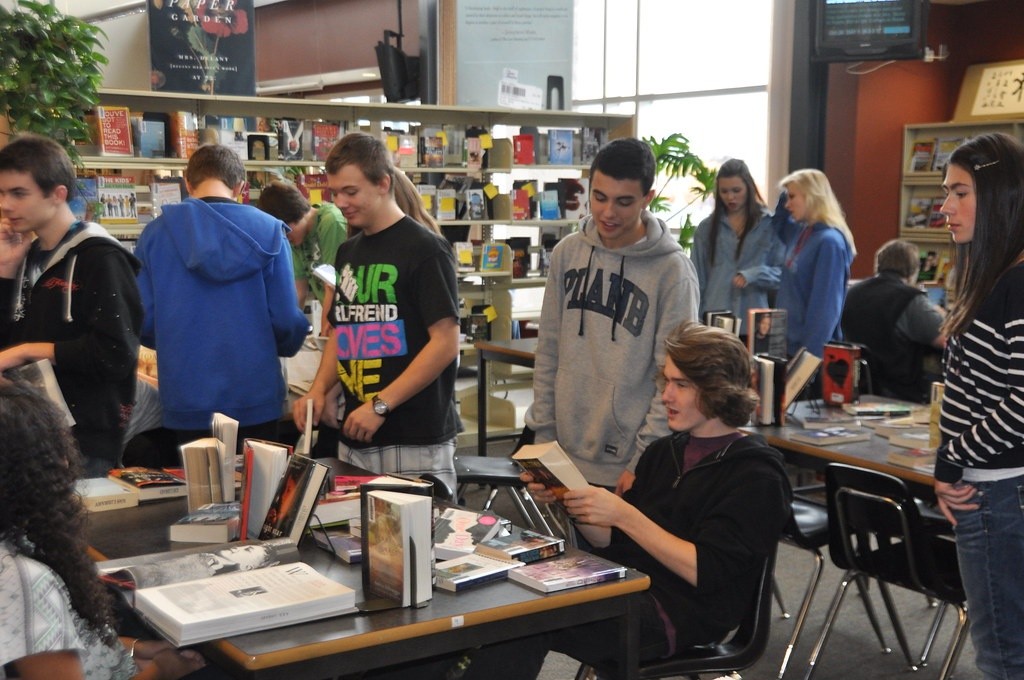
xmin=372 ymin=395 xmax=391 ymax=418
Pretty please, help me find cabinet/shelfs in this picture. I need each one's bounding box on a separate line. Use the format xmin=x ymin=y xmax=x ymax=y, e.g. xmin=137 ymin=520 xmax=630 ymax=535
xmin=899 ymin=117 xmax=1024 ymax=292
xmin=3 ymin=90 xmax=637 ymax=284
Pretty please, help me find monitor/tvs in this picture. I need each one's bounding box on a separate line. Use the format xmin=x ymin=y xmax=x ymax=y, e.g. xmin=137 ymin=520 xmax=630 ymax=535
xmin=374 ymin=41 xmax=421 ymax=103
xmin=814 ymin=0 xmax=923 ymax=55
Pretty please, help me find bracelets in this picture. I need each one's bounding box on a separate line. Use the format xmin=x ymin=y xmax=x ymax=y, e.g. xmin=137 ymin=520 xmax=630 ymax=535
xmin=130 ymin=639 xmax=139 ymax=657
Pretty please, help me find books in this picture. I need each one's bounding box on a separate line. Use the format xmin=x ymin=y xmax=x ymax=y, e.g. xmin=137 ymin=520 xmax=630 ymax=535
xmin=73 ymin=106 xmax=608 ymax=282
xmin=703 ymin=306 xmax=938 ymax=475
xmin=77 ymin=397 xmax=625 ymax=646
xmin=907 ymin=134 xmax=969 ymax=302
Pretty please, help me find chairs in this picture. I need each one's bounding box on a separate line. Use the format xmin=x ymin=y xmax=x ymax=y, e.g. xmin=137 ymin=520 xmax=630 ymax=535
xmin=446 ymin=342 xmax=972 ymax=680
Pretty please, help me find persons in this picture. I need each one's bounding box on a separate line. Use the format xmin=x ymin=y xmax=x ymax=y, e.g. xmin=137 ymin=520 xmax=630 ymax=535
xmin=690 ymin=158 xmax=784 ymax=347
xmin=773 ymin=169 xmax=856 ymax=356
xmin=132 ymin=145 xmax=306 ymax=467
xmin=523 ymin=137 xmax=699 ymax=497
xmin=0 ymin=384 xmax=241 ymax=680
xmin=312 ymin=166 xmax=440 ymax=459
xmin=290 ymin=133 xmax=461 ymax=505
xmin=134 ymin=540 xmax=278 ymax=589
xmin=257 ymin=181 xmax=348 ymax=339
xmin=841 ymin=241 xmax=950 ymax=402
xmin=934 ymin=133 xmax=1024 ymax=680
xmin=0 ymin=136 xmax=140 ymax=473
xmin=362 ymin=320 xmax=794 ymax=680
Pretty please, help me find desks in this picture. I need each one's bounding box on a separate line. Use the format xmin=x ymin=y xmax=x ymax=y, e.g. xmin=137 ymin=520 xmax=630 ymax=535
xmin=68 ymin=462 xmax=654 ymax=679
xmin=746 ymin=392 xmax=944 ymax=483
xmin=473 ymin=337 xmax=539 ymax=457
